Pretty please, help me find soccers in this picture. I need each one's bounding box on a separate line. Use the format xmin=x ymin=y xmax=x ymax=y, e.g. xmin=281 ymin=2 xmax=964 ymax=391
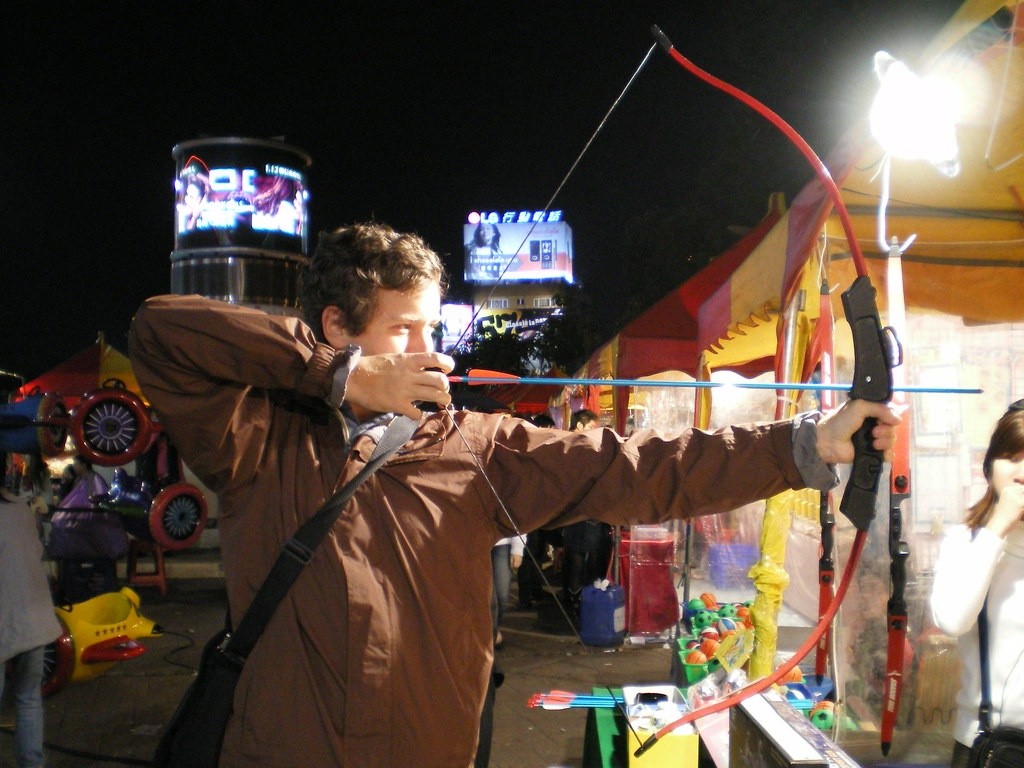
xmin=810 ymin=709 xmax=834 ymax=730
xmin=743 ymin=601 xmax=755 ymax=607
xmin=719 ymin=605 xmax=737 ymax=618
xmin=696 ymin=609 xmax=719 ymax=627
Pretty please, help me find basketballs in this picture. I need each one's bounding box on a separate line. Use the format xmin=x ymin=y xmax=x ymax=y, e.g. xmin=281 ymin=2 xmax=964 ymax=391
xmin=685 ymin=619 xmax=755 ymax=664
xmin=776 ymin=662 xmax=803 ymax=686
xmin=811 ymin=699 xmax=834 ymax=718
xmin=785 ymin=688 xmax=810 ymax=700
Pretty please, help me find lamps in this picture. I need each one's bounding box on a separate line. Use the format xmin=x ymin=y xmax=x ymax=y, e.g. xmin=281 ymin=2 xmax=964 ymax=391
xmin=868 ymin=48 xmax=977 ymax=178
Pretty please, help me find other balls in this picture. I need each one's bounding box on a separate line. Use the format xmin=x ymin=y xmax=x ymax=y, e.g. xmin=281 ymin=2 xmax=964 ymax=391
xmin=690 ymin=592 xmax=718 ymax=609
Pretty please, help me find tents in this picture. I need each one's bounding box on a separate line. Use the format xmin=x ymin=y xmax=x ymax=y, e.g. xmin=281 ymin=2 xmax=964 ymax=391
xmin=490 ymin=1 xmax=1024 ymax=768
xmin=9 ymin=333 xmax=152 ymax=488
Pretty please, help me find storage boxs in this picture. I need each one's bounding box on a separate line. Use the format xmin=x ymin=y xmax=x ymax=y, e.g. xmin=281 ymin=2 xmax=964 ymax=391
xmin=729 ymin=694 xmax=829 ymax=768
xmin=622 ymin=686 xmax=699 ymax=768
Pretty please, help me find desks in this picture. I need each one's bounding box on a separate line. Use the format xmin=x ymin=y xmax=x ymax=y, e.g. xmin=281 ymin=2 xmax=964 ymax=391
xmin=691 ymin=682 xmax=862 ymax=768
xmin=583 ymin=687 xmax=688 ymax=768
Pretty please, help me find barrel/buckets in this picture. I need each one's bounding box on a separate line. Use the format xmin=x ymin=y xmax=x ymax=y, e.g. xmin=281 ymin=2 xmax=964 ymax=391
xmin=581 ymin=580 xmax=625 ymax=647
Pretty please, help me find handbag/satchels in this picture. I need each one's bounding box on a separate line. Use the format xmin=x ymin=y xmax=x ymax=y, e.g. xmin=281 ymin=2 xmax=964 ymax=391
xmin=153 ymin=628 xmax=241 ymax=768
xmin=948 ymin=727 xmax=1024 ymax=768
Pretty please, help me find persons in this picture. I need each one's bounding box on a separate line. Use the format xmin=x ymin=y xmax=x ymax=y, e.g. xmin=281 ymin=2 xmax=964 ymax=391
xmin=825 ymin=558 xmax=921 ymax=730
xmin=9 ymin=455 xmax=123 ymax=594
xmin=1 ymin=460 xmax=71 ymax=768
xmin=249 ymin=177 xmax=301 ymax=236
xmin=126 ymin=219 xmax=897 ymax=768
xmin=517 ymin=416 xmax=559 ymax=611
xmin=561 ymin=408 xmax=608 ymax=625
xmin=488 ymin=534 xmax=526 ymax=649
xmin=465 ymin=224 xmax=502 ymax=281
xmin=928 ymin=406 xmax=1024 ymax=768
